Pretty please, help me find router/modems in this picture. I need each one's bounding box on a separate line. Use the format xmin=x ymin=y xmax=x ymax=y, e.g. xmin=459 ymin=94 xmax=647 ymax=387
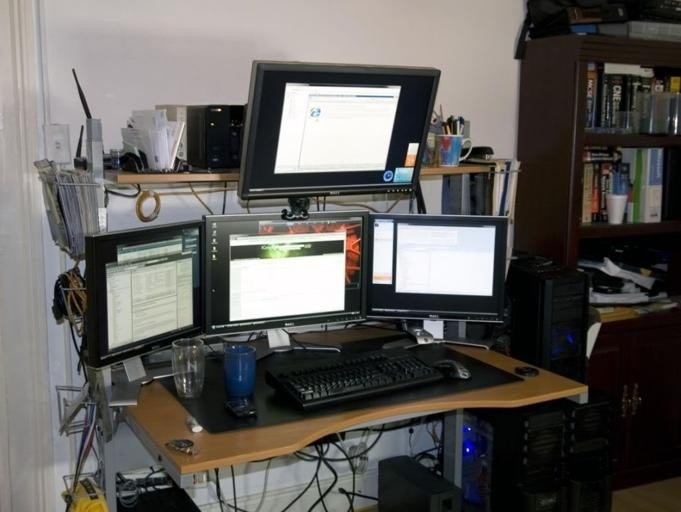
xmin=74 ymin=126 xmax=113 ymax=169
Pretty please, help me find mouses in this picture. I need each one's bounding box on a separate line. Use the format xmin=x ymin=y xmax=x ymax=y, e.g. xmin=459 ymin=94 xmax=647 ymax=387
xmin=430 ymin=359 xmax=473 ymax=380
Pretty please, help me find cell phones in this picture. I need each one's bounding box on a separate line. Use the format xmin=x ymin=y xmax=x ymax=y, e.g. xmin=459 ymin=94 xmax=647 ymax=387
xmin=224 ymin=398 xmax=257 ymax=418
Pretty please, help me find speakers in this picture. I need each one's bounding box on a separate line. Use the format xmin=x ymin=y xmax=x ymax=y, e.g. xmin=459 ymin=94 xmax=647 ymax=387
xmin=186 ymin=103 xmax=246 ymax=173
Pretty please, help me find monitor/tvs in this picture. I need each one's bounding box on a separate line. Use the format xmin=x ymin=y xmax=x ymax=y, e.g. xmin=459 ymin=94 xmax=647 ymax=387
xmin=202 ymin=210 xmax=369 ymax=361
xmin=237 ymin=60 xmax=441 ymax=199
xmin=367 ymin=213 xmax=509 ymax=350
xmin=85 ymin=220 xmax=205 ymax=406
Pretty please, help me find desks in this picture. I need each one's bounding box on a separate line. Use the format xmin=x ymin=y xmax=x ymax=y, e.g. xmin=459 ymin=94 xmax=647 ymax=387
xmin=84 ymin=117 xmax=591 ymax=512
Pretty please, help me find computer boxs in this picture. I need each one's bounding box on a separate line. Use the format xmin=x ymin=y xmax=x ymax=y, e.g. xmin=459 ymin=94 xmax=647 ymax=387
xmin=474 ymin=399 xmax=564 ymax=510
xmin=440 ymin=421 xmax=492 ymax=510
xmin=560 ymin=387 xmax=613 ymax=510
xmin=377 ymin=455 xmax=462 ymax=511
xmin=506 ymin=253 xmax=592 ymax=389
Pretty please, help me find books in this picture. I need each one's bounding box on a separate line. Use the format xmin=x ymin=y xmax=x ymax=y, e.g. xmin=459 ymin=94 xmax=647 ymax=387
xmin=576 ymin=52 xmax=680 ymax=326
xmin=467 ymin=155 xmax=522 ymax=219
xmin=33 ymin=157 xmax=100 ymax=258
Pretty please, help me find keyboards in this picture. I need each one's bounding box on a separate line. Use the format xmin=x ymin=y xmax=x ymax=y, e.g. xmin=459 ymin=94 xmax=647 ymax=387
xmin=266 ymin=350 xmax=445 ymax=410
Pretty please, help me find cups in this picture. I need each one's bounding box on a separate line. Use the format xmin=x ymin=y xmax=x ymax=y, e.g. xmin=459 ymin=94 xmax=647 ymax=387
xmin=603 ymin=192 xmax=629 ymax=225
xmin=222 ymin=344 xmax=257 ymax=399
xmin=168 ymin=337 xmax=208 ymax=399
xmin=432 ymin=131 xmax=473 ymax=167
xmin=613 ymin=108 xmax=641 ymax=136
xmin=633 ymin=91 xmax=672 ymax=136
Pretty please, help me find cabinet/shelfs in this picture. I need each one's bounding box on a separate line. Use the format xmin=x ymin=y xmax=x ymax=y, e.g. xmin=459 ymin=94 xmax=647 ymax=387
xmin=510 ymin=33 xmax=680 ymax=495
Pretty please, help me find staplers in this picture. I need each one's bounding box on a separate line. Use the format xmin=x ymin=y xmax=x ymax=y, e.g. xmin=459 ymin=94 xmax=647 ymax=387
xmin=459 ymin=147 xmax=496 ymax=165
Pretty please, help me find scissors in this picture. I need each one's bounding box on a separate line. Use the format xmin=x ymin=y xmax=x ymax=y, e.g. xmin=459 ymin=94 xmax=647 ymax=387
xmin=430 ymin=105 xmax=442 ymax=128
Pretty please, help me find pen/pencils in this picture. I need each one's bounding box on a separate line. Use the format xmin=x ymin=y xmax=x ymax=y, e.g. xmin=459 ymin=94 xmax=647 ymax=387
xmin=441 ymin=116 xmax=464 ymax=135
